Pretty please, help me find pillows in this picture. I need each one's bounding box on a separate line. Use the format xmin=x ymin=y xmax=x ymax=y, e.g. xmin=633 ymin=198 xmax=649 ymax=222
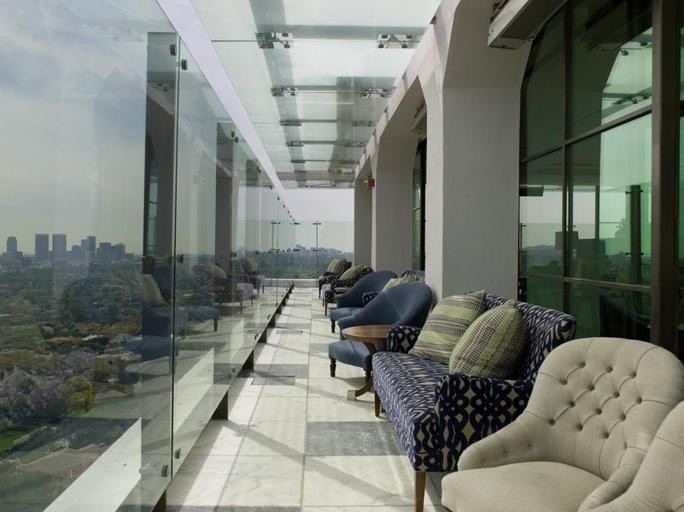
xmin=407 ymin=288 xmax=530 ymax=379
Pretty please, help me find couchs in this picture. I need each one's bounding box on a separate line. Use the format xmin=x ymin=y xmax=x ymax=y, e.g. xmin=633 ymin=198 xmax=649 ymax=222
xmin=369 ymin=288 xmax=577 ymax=512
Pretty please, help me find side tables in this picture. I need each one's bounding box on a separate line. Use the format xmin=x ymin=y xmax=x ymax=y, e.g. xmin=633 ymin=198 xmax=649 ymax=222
xmin=342 ymin=323 xmax=394 ymax=417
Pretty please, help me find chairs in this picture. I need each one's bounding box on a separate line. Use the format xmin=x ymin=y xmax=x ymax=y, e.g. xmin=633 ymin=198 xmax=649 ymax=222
xmin=316 ymin=256 xmax=434 ymax=383
xmin=579 ymin=401 xmax=684 ymax=512
xmin=440 ymin=338 xmax=684 ymax=512
